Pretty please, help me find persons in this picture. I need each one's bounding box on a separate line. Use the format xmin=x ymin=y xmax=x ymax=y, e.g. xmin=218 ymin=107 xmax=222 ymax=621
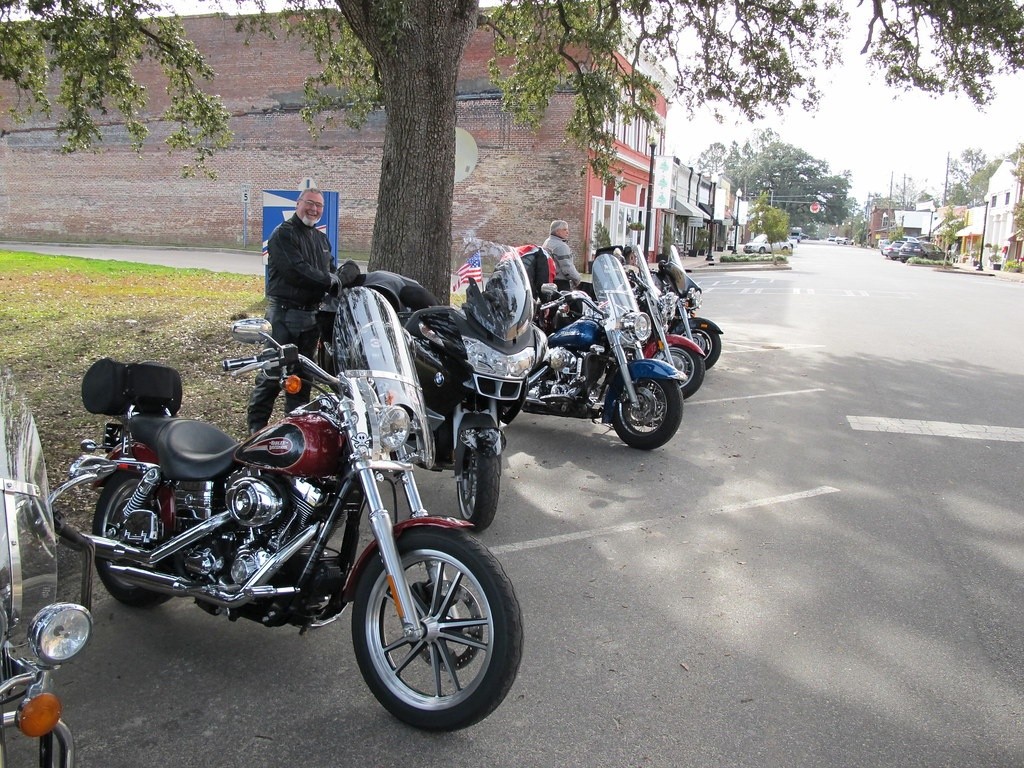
xmin=248 ymin=188 xmax=361 ymax=440
xmin=541 ymin=220 xmax=581 ymax=321
xmin=349 ymin=270 xmax=439 ymax=326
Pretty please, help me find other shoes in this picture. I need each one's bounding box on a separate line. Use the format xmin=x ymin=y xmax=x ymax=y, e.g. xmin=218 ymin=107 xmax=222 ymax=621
xmin=247 ymin=428 xmax=258 ymax=437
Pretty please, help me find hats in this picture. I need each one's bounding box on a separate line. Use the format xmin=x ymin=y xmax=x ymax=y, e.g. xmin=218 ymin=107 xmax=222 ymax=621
xmin=334 ymin=260 xmax=366 ymax=287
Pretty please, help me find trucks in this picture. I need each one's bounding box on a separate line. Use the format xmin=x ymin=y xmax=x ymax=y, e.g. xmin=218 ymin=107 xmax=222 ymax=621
xmin=790 ymin=227 xmax=801 ymax=242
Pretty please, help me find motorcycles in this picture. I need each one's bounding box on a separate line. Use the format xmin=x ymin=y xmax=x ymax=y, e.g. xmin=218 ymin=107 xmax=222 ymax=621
xmin=0 ymin=371 xmax=92 ymax=768
xmin=16 ymin=280 xmax=524 ymax=731
xmin=503 ymin=244 xmax=724 ymax=450
xmin=313 ymin=240 xmax=549 ymax=533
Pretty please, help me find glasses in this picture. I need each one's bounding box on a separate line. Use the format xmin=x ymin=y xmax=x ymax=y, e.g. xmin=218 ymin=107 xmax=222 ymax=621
xmin=299 ymin=199 xmax=325 ymax=208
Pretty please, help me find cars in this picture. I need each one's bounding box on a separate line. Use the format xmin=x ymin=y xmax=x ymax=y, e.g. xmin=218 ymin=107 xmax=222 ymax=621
xmin=836 ymin=237 xmax=854 ymax=245
xmin=743 ymin=232 xmax=793 ymax=254
xmin=888 ymin=241 xmax=906 ymax=259
xmin=879 ymin=237 xmax=919 ymax=256
xmin=898 ymin=241 xmax=950 ymax=263
xmin=829 ymin=235 xmax=835 ymax=240
xmin=787 ymin=236 xmax=797 ymax=248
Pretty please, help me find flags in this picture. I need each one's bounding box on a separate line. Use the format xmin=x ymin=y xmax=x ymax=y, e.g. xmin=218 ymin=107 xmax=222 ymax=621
xmin=453 ymin=252 xmax=481 ymax=291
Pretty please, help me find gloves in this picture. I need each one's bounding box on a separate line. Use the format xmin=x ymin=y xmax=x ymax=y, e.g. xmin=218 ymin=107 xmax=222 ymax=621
xmin=329 ymin=272 xmax=343 ymax=297
xmin=572 ymin=274 xmax=581 ymax=287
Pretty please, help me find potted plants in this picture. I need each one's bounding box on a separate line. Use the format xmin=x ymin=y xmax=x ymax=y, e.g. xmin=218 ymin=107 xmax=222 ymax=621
xmin=629 ymin=222 xmax=644 ymax=231
xmin=690 ymin=229 xmax=733 ymax=257
xmin=657 ymin=225 xmax=675 ymax=262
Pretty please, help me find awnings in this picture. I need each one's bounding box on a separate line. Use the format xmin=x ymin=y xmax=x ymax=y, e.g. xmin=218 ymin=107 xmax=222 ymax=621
xmin=699 ymin=202 xmax=712 ymax=216
xmin=676 ymin=200 xmax=700 ymax=216
xmin=690 ymin=203 xmax=710 ymax=220
xmin=730 ymin=213 xmax=745 ymax=226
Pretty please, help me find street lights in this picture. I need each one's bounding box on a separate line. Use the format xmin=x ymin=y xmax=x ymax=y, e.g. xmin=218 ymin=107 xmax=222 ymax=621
xmin=928 ymin=204 xmax=936 ymax=242
xmin=706 ymin=171 xmax=718 ymax=261
xmin=975 ymin=194 xmax=992 ymax=271
xmin=643 ymin=129 xmax=658 ymax=262
xmin=732 ymin=187 xmax=742 ymax=254
xmin=901 ymin=210 xmax=906 ymax=238
